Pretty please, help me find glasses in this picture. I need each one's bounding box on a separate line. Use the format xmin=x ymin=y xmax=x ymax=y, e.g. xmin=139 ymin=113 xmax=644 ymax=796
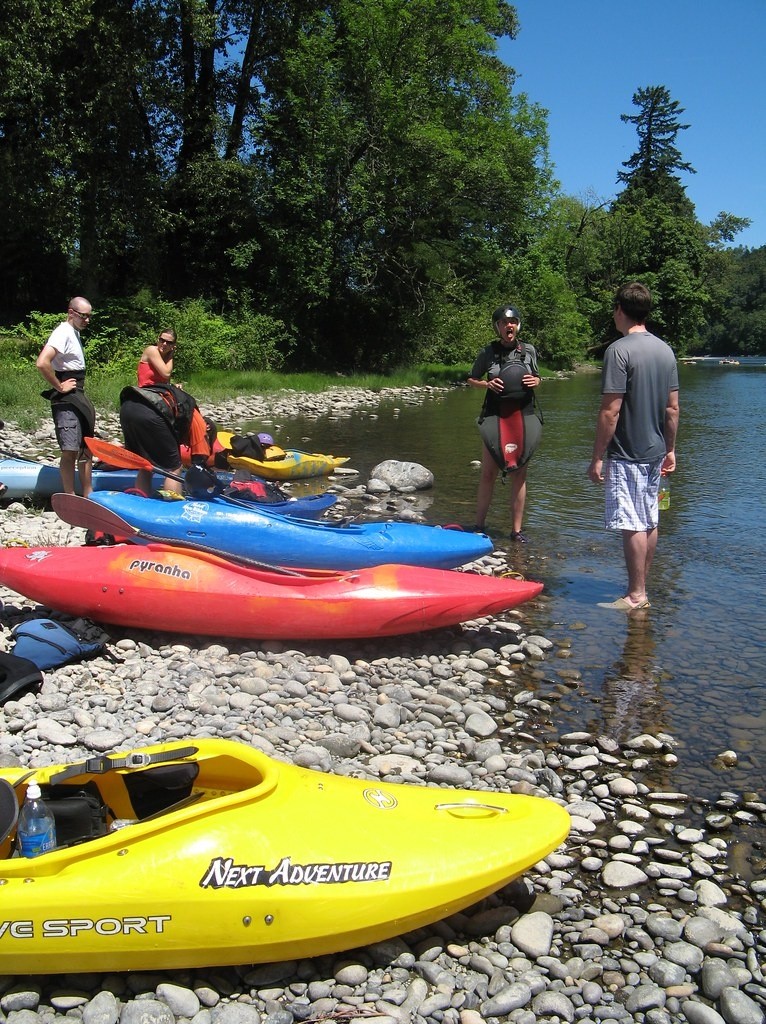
xmin=73 ymin=309 xmax=92 ymax=319
xmin=159 ymin=338 xmax=174 ymax=345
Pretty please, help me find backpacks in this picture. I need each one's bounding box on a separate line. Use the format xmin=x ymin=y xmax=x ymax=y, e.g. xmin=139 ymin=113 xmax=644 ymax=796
xmin=490 ymin=340 xmax=531 ymax=402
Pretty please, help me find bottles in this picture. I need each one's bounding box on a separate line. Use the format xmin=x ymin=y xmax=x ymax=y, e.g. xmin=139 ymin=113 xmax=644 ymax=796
xmin=17 ymin=780 xmax=56 ymax=858
xmin=657 ymin=476 xmax=671 ymax=510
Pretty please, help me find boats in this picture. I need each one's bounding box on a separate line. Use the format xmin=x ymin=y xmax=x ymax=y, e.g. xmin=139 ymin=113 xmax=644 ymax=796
xmin=228 ymin=446 xmax=351 ymax=482
xmin=84 ymin=486 xmax=493 ymax=574
xmin=0 ymin=542 xmax=545 ymax=647
xmin=0 ymin=736 xmax=572 ymax=977
xmin=1 ymin=455 xmax=267 ymax=502
xmin=183 ymin=471 xmax=338 ymax=521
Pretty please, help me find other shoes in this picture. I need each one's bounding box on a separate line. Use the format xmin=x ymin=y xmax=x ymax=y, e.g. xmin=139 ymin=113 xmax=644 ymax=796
xmin=472 ymin=525 xmax=487 ymax=535
xmin=511 ymin=528 xmax=531 ymax=543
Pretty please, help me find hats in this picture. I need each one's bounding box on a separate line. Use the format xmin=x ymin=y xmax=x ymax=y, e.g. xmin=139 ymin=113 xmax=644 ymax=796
xmin=202 ymin=416 xmax=217 ymax=456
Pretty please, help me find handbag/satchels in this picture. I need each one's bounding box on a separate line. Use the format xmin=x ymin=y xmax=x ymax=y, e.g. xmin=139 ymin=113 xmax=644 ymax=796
xmin=19 ymin=791 xmax=106 ymax=846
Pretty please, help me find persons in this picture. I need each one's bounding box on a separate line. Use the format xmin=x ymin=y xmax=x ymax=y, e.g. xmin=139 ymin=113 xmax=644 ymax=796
xmin=119 ymin=383 xmax=217 ymax=498
xmin=587 ymin=281 xmax=680 ymax=612
xmin=36 ymin=297 xmax=96 ymax=497
xmin=137 ymin=329 xmax=177 ymax=388
xmin=466 ymin=305 xmax=542 ymax=543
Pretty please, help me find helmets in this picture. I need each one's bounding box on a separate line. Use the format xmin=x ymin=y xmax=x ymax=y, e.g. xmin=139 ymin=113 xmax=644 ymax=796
xmin=184 ymin=463 xmax=224 ymax=499
xmin=492 ymin=306 xmax=521 ymax=338
xmin=257 ymin=432 xmax=286 ymax=461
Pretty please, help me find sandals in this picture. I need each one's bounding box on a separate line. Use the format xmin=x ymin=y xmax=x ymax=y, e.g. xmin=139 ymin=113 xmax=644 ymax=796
xmin=596 ymin=598 xmax=652 ymax=609
